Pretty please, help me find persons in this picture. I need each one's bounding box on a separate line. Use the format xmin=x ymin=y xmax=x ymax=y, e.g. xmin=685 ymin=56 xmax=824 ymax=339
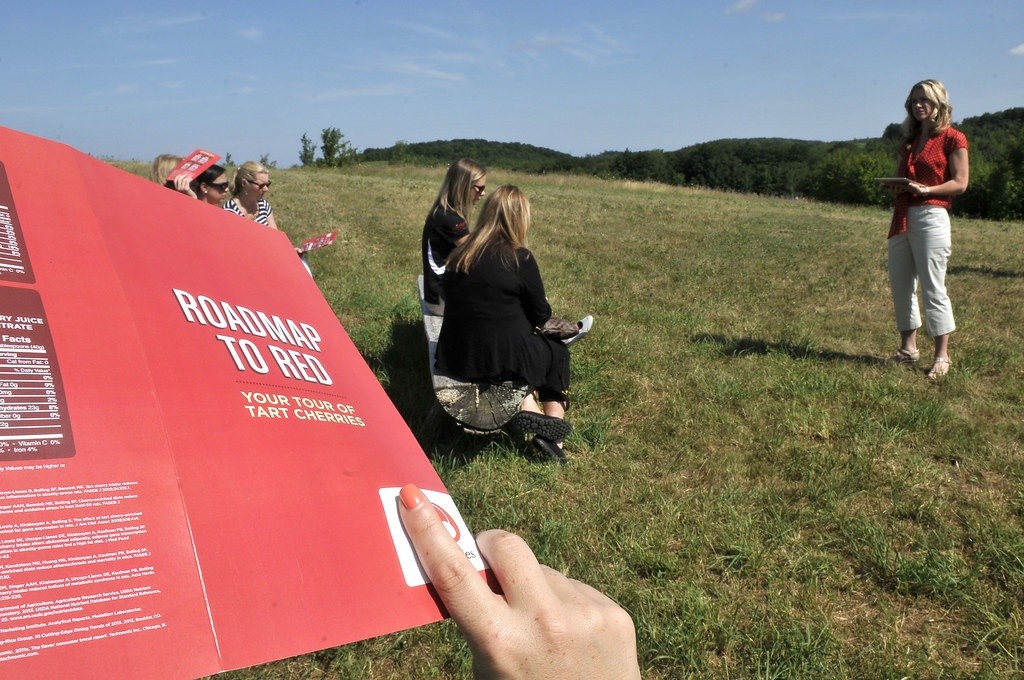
xmin=435 ymin=184 xmax=571 ymax=464
xmin=888 ymin=79 xmax=969 ymax=378
xmin=422 ymin=158 xmax=593 ymax=346
xmin=398 ymin=484 xmax=642 ymax=680
xmin=222 ymin=161 xmax=311 ymax=276
xmin=149 ymin=154 xmax=228 ymax=207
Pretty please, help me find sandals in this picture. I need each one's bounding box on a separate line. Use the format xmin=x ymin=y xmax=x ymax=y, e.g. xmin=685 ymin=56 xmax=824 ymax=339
xmin=890 ymin=347 xmax=919 ymax=363
xmin=926 ymin=355 xmax=952 ymax=379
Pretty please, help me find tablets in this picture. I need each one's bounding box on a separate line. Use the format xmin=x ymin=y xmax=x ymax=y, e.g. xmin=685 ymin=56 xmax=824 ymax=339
xmin=873 ymin=177 xmax=927 ymax=189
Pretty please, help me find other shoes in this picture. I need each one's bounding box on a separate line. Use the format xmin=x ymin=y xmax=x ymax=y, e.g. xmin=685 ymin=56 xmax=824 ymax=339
xmin=561 ymin=314 xmax=592 ymax=347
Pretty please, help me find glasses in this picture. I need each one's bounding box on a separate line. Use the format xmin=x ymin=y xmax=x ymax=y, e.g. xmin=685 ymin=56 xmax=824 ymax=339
xmin=247 ymin=179 xmax=272 ymax=189
xmin=206 ymin=181 xmax=229 ymax=192
xmin=473 ymin=185 xmax=485 ymax=194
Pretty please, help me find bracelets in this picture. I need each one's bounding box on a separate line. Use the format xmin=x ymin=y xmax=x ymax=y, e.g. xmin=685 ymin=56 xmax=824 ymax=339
xmin=923 ymin=187 xmax=928 ymax=196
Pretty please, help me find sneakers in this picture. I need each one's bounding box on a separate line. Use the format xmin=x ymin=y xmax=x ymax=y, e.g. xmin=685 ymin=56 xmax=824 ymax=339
xmin=532 ymin=433 xmax=568 ymax=467
xmin=514 ymin=410 xmax=572 ymax=439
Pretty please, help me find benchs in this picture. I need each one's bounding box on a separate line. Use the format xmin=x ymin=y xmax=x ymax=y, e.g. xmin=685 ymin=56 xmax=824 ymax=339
xmin=416 ymin=274 xmax=526 ymax=432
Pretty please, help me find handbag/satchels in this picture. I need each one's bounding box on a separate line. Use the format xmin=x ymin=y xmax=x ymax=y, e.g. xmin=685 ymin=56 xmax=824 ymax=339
xmin=533 ymin=317 xmax=580 ymax=339
